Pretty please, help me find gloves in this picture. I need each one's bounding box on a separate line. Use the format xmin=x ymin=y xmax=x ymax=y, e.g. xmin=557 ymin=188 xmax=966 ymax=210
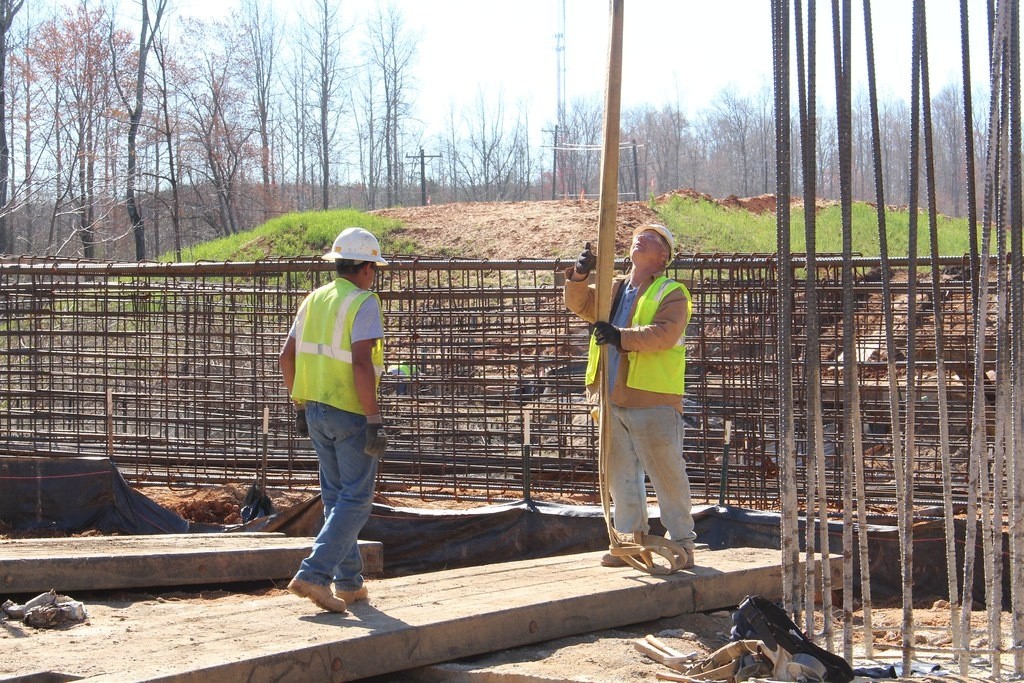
xmin=593 ymin=321 xmax=620 ymax=348
xmin=293 ymin=404 xmax=311 ymax=437
xmin=573 ymin=242 xmax=596 ymax=274
xmin=363 ymin=412 xmax=388 ymax=461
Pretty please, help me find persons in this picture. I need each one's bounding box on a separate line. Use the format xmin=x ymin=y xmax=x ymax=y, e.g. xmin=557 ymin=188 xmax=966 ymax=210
xmin=386 ymin=360 xmax=426 ymax=396
xmin=563 ymin=224 xmax=697 ymax=571
xmin=278 ymin=227 xmax=388 ymax=614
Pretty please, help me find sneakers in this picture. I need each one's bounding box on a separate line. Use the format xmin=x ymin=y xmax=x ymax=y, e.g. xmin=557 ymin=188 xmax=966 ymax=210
xmin=333 ymin=584 xmax=368 ymax=604
xmin=601 ymin=545 xmax=653 ymax=567
xmin=288 ymin=573 xmax=346 ymax=611
xmin=671 ymin=548 xmax=695 ymax=569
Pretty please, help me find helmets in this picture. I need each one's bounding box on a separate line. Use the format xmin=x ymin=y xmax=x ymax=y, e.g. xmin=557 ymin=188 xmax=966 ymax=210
xmin=633 ymin=224 xmax=675 ymax=267
xmin=322 ymin=226 xmax=387 ymax=266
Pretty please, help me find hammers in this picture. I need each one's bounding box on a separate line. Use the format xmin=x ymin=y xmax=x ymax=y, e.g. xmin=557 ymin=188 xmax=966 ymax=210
xmin=644 ymin=633 xmax=698 ymax=661
